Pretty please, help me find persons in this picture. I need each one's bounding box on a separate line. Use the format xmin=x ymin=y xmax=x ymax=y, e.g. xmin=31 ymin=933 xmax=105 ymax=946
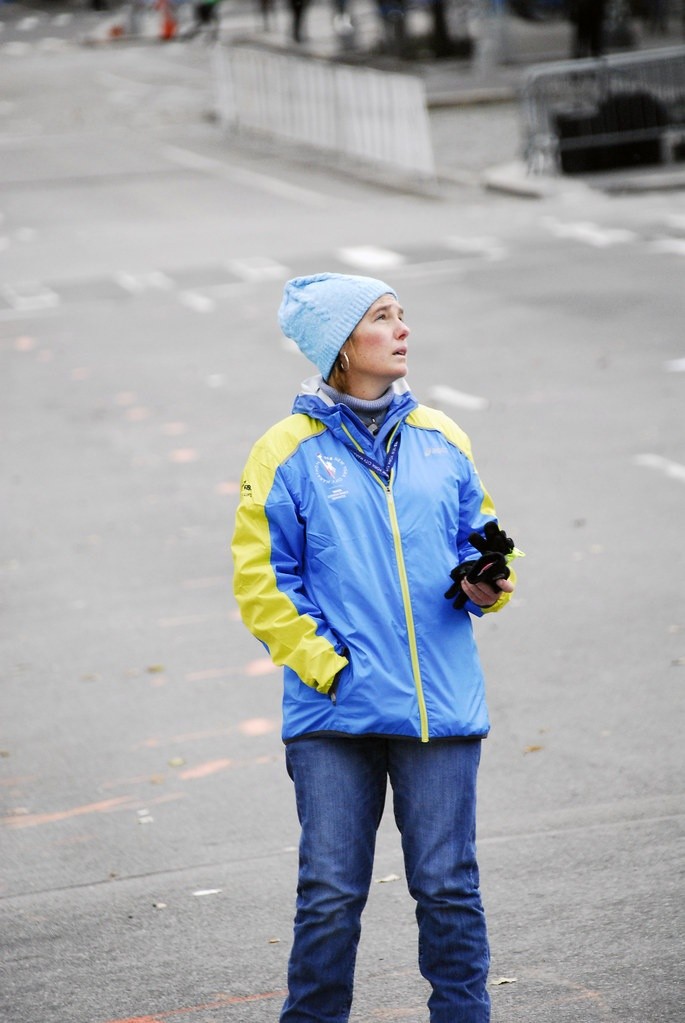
xmin=234 ymin=273 xmax=519 ymax=1023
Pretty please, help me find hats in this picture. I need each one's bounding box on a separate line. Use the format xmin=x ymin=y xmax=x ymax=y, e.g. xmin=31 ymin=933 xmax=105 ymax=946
xmin=276 ymin=272 xmax=397 ymax=382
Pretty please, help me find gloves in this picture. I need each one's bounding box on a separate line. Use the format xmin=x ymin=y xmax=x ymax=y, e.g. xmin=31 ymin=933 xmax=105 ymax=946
xmin=444 ymin=522 xmax=515 ymax=609
